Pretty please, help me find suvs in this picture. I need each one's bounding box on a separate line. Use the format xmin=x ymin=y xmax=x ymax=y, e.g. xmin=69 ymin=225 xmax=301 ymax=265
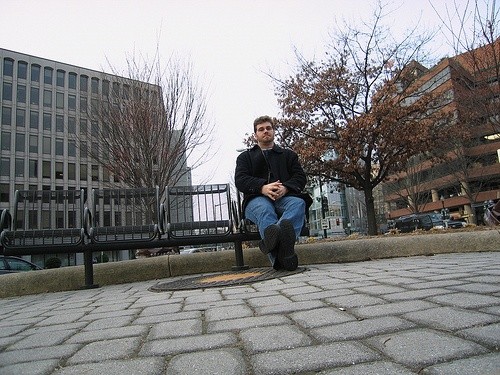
xmin=362 ymin=214 xmax=469 ymax=236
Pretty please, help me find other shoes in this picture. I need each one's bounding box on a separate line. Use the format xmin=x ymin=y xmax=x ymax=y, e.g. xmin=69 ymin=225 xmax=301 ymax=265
xmin=258 ymin=223 xmax=281 ymax=255
xmin=271 ymin=218 xmax=299 ymax=271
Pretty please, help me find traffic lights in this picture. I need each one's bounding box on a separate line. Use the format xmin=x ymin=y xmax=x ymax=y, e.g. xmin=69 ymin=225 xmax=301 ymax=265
xmin=336 ymin=218 xmax=340 ymax=225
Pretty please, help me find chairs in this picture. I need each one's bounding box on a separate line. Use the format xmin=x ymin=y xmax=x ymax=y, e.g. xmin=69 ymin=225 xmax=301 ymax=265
xmin=0 ymin=183 xmax=309 ymax=290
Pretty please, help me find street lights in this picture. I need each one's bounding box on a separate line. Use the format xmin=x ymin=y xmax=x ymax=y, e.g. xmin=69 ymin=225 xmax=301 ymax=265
xmin=440 ymin=196 xmax=445 ymax=228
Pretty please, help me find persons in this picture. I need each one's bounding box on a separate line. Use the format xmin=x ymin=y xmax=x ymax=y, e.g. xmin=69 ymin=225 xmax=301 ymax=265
xmin=235 ymin=116 xmax=313 ymax=272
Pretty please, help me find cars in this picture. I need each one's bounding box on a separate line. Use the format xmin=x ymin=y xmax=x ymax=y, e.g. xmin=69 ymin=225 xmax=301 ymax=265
xmin=0 ymin=255 xmax=45 ymax=276
xmin=178 ymin=247 xmax=222 ymax=255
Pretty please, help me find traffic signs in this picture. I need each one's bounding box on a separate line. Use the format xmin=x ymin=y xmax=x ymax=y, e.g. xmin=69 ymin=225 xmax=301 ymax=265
xmin=321 ymin=219 xmax=330 ymax=229
